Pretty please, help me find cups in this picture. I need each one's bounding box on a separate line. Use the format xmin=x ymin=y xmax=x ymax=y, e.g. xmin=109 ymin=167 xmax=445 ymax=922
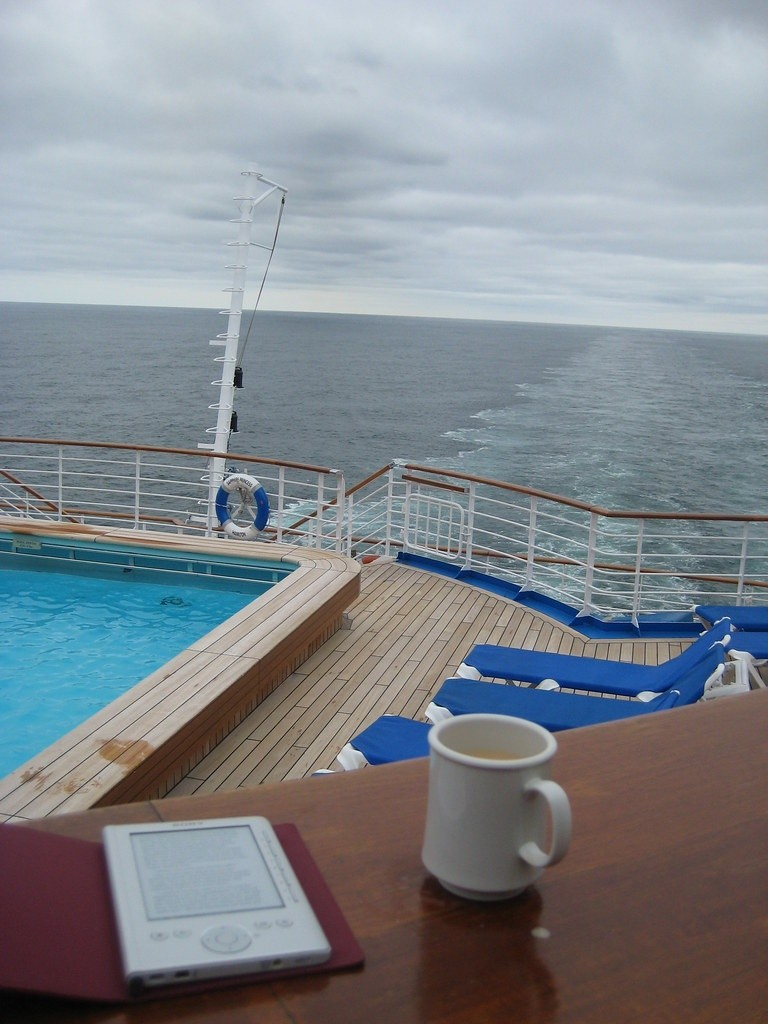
xmin=421 ymin=714 xmax=572 ymax=902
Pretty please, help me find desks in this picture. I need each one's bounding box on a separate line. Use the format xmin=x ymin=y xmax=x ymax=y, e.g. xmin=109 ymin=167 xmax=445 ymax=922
xmin=0 ymin=685 xmax=768 ymax=1024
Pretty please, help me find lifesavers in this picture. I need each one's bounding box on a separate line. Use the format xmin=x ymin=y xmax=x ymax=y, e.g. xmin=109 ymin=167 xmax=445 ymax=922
xmin=214 ymin=473 xmax=270 ymax=541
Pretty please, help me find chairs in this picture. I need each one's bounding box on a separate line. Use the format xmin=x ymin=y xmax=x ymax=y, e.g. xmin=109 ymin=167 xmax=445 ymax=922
xmin=336 ymin=714 xmax=432 ymax=771
xmin=457 ymin=615 xmax=740 ymax=700
xmin=424 ymin=639 xmax=748 ymax=723
